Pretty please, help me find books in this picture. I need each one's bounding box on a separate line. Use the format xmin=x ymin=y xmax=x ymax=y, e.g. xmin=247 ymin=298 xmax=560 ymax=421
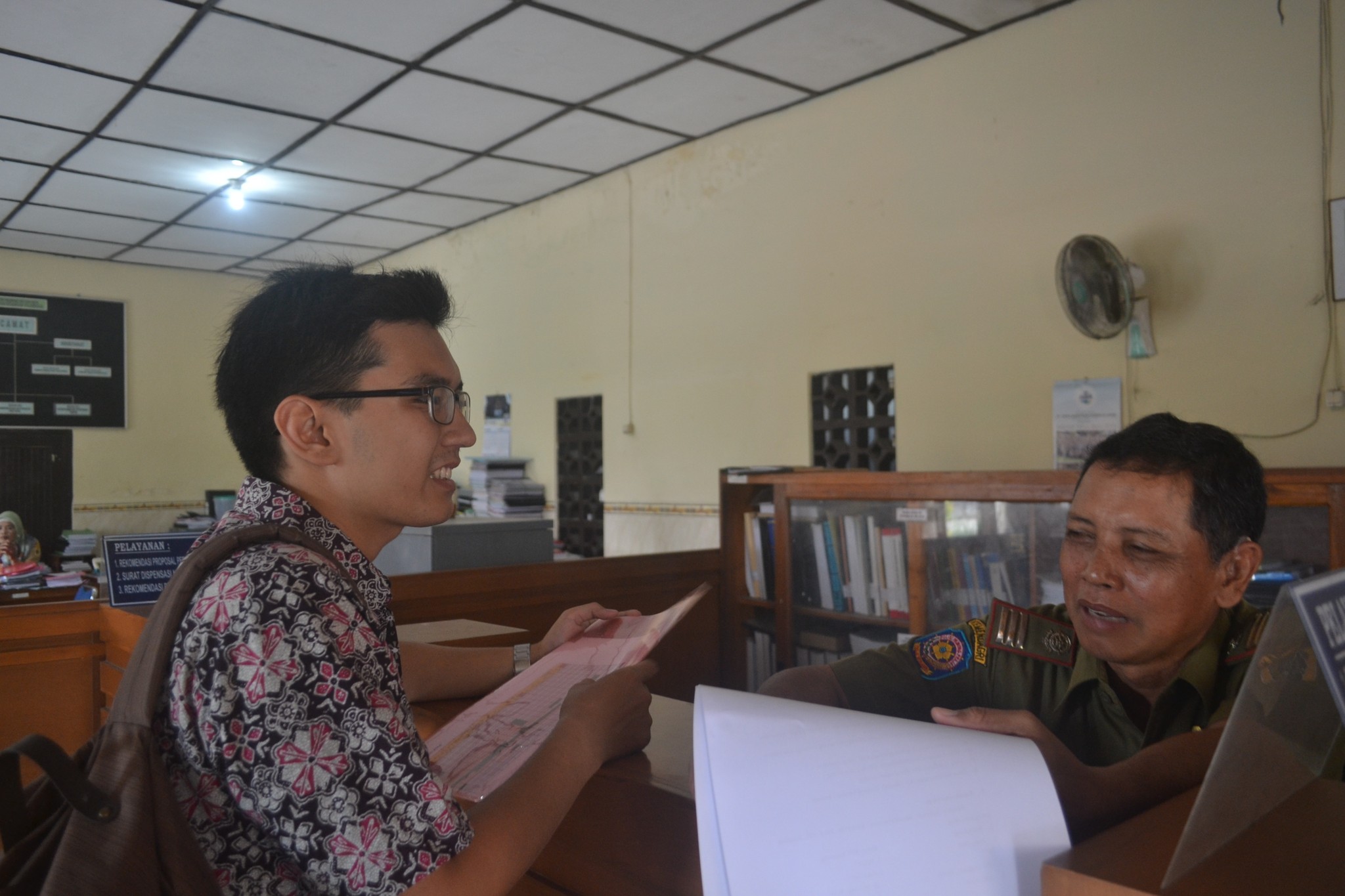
xmin=53 ymin=528 xmax=98 ymax=557
xmin=456 ymin=453 xmax=550 ymax=520
xmin=0 ymin=561 xmax=91 ymax=591
xmin=168 ymin=511 xmax=215 ymax=532
xmin=739 ymin=499 xmax=1028 ymax=692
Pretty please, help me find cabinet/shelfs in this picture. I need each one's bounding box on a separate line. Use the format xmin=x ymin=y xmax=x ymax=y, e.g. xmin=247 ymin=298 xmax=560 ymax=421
xmin=712 ymin=462 xmax=1344 ymax=698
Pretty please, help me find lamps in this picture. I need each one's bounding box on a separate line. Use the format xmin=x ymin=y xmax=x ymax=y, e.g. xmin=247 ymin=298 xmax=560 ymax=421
xmin=225 ymin=176 xmax=247 ymax=212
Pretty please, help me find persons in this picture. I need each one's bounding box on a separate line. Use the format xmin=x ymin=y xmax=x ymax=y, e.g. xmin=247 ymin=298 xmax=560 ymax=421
xmin=753 ymin=408 xmax=1274 ymax=847
xmin=0 ymin=510 xmax=41 ymax=567
xmin=148 ymin=258 xmax=657 ymax=896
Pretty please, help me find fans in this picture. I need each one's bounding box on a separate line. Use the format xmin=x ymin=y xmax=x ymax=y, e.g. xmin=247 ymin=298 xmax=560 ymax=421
xmin=1054 ymin=233 xmax=1157 ymax=361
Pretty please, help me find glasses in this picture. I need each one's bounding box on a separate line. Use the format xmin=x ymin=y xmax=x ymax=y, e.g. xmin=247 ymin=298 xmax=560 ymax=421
xmin=273 ymin=385 xmax=471 ymax=426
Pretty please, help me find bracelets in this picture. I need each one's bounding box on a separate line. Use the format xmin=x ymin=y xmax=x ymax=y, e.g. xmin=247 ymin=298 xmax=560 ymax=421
xmin=512 ymin=642 xmax=531 ymax=676
